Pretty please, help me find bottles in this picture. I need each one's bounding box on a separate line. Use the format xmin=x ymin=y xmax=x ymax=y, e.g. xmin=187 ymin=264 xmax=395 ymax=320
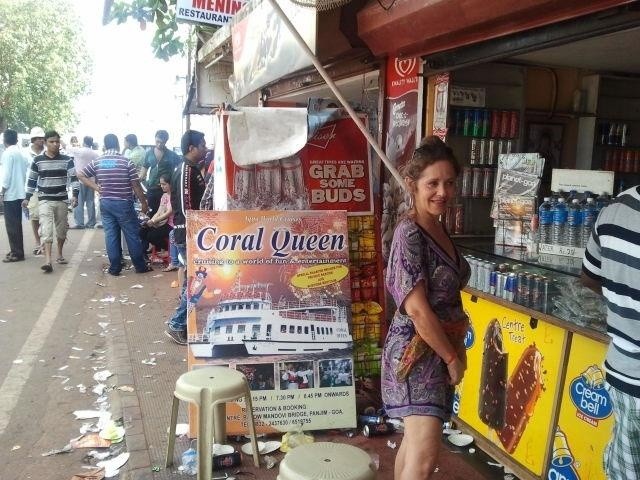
xmin=566 ymin=190 xmax=579 ymax=208
xmin=539 ymin=197 xmax=553 ymax=244
xmin=582 ymin=191 xmax=592 ymax=206
xmin=552 ymin=190 xmax=562 ymax=207
xmin=580 ymin=198 xmax=597 ymax=249
xmin=180 ymin=448 xmax=198 ymax=474
xmin=553 ymin=198 xmax=569 ymax=245
xmin=568 ymin=199 xmax=582 ymax=247
xmin=596 ymin=192 xmax=609 ymax=206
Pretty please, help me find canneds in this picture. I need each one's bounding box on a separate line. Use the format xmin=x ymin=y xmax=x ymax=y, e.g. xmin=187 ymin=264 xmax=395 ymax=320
xmin=469 ymin=139 xmax=515 ymax=166
xmin=441 ymin=204 xmax=466 ymax=235
xmin=233 ymin=164 xmax=256 ymax=211
xmin=596 ymin=117 xmax=640 ymax=193
xmin=364 ymin=422 xmax=391 ymax=436
xmin=444 ymin=107 xmax=521 ymax=138
xmin=456 ymin=167 xmax=499 ymax=197
xmin=359 ymin=414 xmax=383 ymax=424
xmin=283 ymin=156 xmax=304 ymax=203
xmin=463 ymin=254 xmax=557 ymax=314
xmin=256 ymin=159 xmax=281 ymax=207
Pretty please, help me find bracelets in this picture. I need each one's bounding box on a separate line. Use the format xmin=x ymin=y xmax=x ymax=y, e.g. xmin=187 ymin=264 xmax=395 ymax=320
xmin=447 ymin=354 xmax=460 ymax=367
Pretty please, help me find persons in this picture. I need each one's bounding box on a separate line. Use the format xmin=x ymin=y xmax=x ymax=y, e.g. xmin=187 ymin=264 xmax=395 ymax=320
xmin=144 ymin=172 xmax=173 ymax=260
xmin=138 ymin=129 xmax=181 ymax=220
xmin=580 ymin=183 xmax=640 ymax=476
xmin=19 ymin=130 xmax=80 ymax=273
xmin=122 ymin=133 xmax=147 ymax=205
xmin=163 ymin=129 xmax=211 ymax=347
xmin=70 ymin=134 xmax=99 ymax=229
xmin=20 ymin=126 xmax=47 ymax=256
xmin=77 ymin=133 xmax=154 ymax=276
xmin=378 ymin=135 xmax=470 ymax=477
xmin=1 ymin=128 xmax=30 ymax=263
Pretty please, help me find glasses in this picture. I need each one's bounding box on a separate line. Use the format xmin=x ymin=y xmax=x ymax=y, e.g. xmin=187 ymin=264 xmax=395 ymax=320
xmin=411 ymin=146 xmax=454 ymax=161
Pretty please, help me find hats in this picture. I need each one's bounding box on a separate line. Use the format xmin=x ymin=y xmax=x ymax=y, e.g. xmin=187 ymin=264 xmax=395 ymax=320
xmin=30 ymin=126 xmax=46 ymax=138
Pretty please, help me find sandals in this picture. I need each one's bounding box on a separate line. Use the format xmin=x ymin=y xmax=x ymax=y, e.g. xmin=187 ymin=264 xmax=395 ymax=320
xmin=162 ymin=265 xmax=177 ymax=272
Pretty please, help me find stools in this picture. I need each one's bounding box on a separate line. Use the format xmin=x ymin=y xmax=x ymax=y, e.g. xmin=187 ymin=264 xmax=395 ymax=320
xmin=279 ymin=440 xmax=376 ymax=480
xmin=166 ymin=365 xmax=260 ymax=479
xmin=148 ymin=238 xmax=171 ymax=267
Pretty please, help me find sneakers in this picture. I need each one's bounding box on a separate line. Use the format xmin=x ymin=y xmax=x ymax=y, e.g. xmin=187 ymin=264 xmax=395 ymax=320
xmin=3 ymin=255 xmax=21 ymax=262
xmin=55 ymin=257 xmax=66 ymax=265
xmin=42 ymin=263 xmax=53 ymax=271
xmin=164 ymin=328 xmax=188 ymax=346
